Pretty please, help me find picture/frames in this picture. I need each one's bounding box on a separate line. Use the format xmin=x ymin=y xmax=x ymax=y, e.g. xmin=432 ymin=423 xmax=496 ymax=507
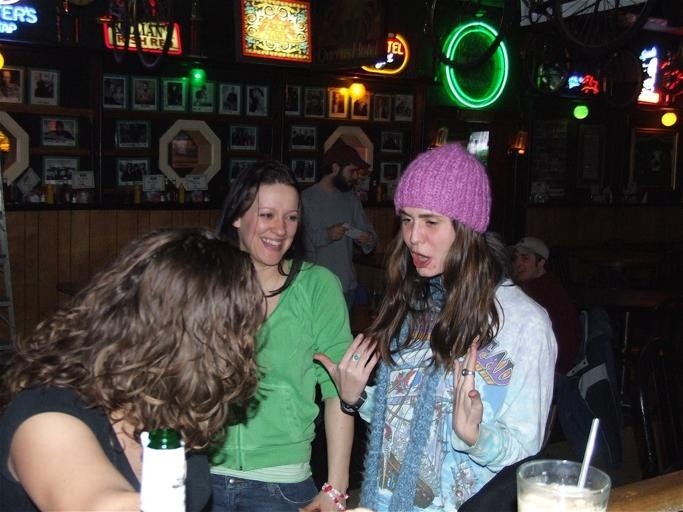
xmin=629 ymin=128 xmax=680 ymax=192
xmin=350 ymin=91 xmax=372 ymax=120
xmin=326 ymin=87 xmax=350 ymax=120
xmin=372 ymin=93 xmax=393 ymax=121
xmin=190 ymin=79 xmax=217 ymax=113
xmin=227 ymin=157 xmax=260 ymax=187
xmin=393 ymin=93 xmax=415 ymax=123
xmin=41 ymin=115 xmax=79 ymax=150
xmin=283 ymin=83 xmax=304 ymax=116
xmin=245 ymin=85 xmax=270 ymax=117
xmin=44 ymin=156 xmax=81 ymax=186
xmin=70 ymin=170 xmax=96 ymax=190
xmin=112 ymin=118 xmax=152 ymax=151
xmin=288 ymin=124 xmax=318 ymax=153
xmin=217 ymin=81 xmax=244 ymax=115
xmin=132 ymin=75 xmax=160 ymax=112
xmin=380 ymin=130 xmax=405 ymax=153
xmin=102 ymin=73 xmax=130 ymax=112
xmin=160 ymin=77 xmax=190 ymax=114
xmin=0 ymin=66 xmax=26 ymax=103
xmin=288 ymin=157 xmax=318 ymax=184
xmin=114 ymin=156 xmax=153 ymax=188
xmin=302 ymin=86 xmax=327 ymax=118
xmin=378 ymin=160 xmax=404 ymax=185
xmin=29 ymin=67 xmax=60 ymax=106
xmin=225 ymin=124 xmax=260 ymax=154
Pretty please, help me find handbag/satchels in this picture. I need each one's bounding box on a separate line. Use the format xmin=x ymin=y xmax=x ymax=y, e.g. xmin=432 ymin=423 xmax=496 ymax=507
xmin=557 ymin=338 xmax=623 ymax=468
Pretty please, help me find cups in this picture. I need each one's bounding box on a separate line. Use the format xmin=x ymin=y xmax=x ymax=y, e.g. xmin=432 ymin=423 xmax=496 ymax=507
xmin=513 ymin=460 xmax=611 ymax=512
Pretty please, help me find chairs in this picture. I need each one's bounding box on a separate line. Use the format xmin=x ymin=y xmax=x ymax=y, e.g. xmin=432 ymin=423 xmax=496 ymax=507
xmin=641 ymin=298 xmax=683 ymax=342
xmin=634 ymin=334 xmax=683 ymax=479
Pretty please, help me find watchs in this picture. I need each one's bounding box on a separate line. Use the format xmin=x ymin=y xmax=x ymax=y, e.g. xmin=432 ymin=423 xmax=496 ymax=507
xmin=340 ymin=391 xmax=367 ymax=416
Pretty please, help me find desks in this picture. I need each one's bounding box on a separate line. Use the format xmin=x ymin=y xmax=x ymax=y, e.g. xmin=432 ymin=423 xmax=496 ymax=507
xmin=606 ymin=469 xmax=683 ymax=512
xmin=608 ymin=287 xmax=670 ymax=408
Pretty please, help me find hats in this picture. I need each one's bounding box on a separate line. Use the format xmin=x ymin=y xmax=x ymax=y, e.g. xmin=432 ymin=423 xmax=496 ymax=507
xmin=325 ymin=144 xmax=370 ymax=169
xmin=513 ymin=237 xmax=549 ymax=260
xmin=393 ymin=142 xmax=492 ymax=233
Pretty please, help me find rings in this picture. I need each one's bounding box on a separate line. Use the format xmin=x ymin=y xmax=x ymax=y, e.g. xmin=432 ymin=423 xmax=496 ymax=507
xmin=461 ymin=369 xmax=475 ymax=376
xmin=352 ymin=354 xmax=360 ymax=362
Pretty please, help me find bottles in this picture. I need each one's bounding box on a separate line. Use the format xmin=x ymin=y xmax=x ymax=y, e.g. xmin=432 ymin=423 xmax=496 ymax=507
xmin=138 ymin=427 xmax=184 ymax=512
xmin=43 ymin=179 xmax=185 ymax=205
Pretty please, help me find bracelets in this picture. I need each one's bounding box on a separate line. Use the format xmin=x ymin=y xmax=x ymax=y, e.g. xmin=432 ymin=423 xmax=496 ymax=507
xmin=321 ymin=485 xmax=345 ymax=511
xmin=325 ymin=482 xmax=349 ymax=500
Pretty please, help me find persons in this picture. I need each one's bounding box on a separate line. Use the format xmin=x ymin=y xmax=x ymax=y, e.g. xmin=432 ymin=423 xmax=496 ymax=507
xmin=507 ymin=236 xmax=579 ymax=371
xmin=204 ymin=159 xmax=354 ymax=512
xmin=0 ymin=227 xmax=263 ymax=512
xmin=0 ymin=70 xmax=411 ymax=184
xmin=294 ymin=144 xmax=378 ymax=330
xmin=312 ymin=142 xmax=558 ymax=512
